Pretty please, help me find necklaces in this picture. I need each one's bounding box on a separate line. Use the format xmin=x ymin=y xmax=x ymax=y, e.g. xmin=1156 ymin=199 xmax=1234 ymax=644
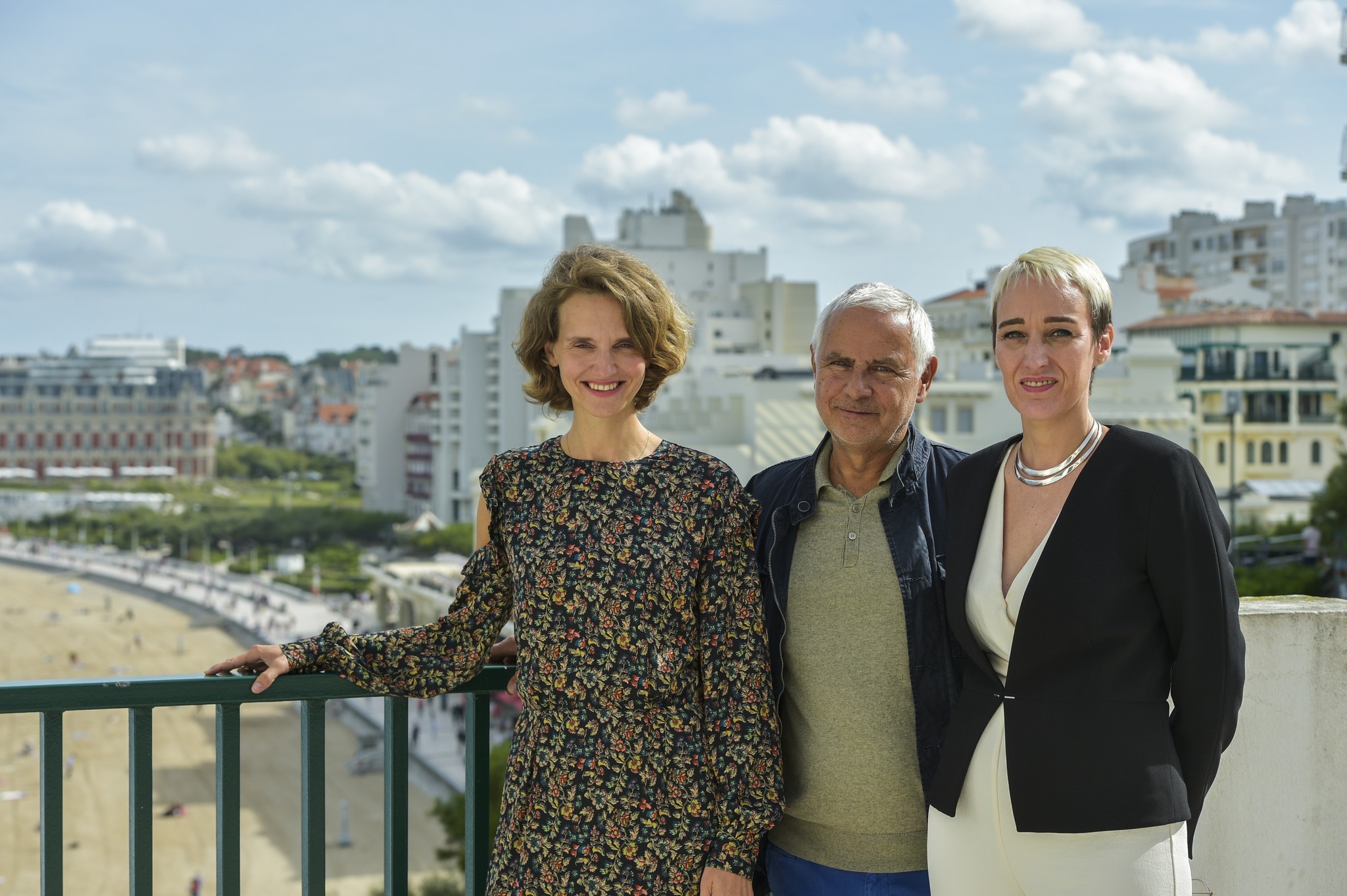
xmin=1014 ymin=418 xmax=1102 ymax=487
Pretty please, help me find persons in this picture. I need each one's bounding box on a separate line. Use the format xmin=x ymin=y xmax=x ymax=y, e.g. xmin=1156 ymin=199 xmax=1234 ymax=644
xmin=926 ymin=245 xmax=1246 ymax=896
xmin=748 ymin=277 xmax=973 ymax=896
xmin=204 ymin=241 xmax=784 ymax=896
